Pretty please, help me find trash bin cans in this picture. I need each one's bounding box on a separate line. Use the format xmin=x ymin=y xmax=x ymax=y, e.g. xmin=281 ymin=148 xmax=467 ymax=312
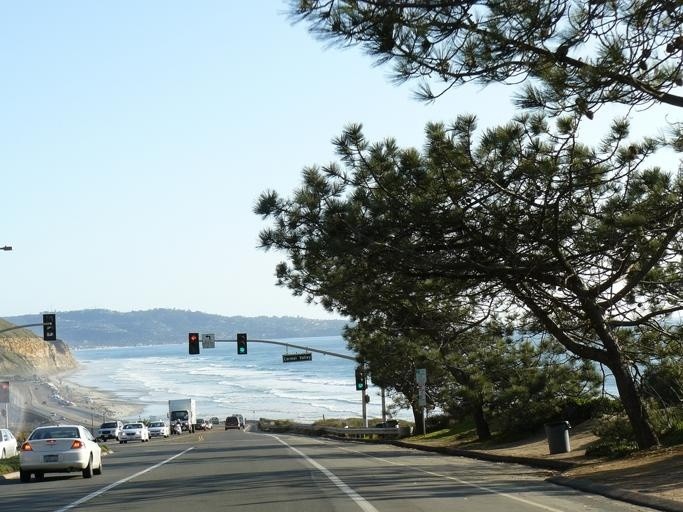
xmin=376 ymin=420 xmax=400 ymax=438
xmin=544 ymin=420 xmax=571 ymax=454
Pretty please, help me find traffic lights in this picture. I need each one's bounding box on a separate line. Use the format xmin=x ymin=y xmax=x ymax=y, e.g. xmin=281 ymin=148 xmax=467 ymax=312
xmin=188 ymin=332 xmax=199 ymax=354
xmin=237 ymin=332 xmax=248 ymax=354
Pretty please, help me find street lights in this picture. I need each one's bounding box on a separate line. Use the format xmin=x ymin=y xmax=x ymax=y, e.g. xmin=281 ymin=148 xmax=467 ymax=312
xmin=355 ymin=368 xmax=366 ymax=390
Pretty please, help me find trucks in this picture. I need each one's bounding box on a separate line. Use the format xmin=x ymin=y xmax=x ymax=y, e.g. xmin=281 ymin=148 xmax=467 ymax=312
xmin=169 ymin=399 xmax=195 ymax=433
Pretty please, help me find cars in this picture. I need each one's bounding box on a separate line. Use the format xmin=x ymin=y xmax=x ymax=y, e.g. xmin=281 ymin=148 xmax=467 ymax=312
xmin=0 ymin=429 xmax=18 ymax=460
xmin=18 ymin=425 xmax=102 ymax=482
xmin=196 ymin=414 xmax=245 ymax=431
xmin=95 ymin=420 xmax=169 ymax=444
